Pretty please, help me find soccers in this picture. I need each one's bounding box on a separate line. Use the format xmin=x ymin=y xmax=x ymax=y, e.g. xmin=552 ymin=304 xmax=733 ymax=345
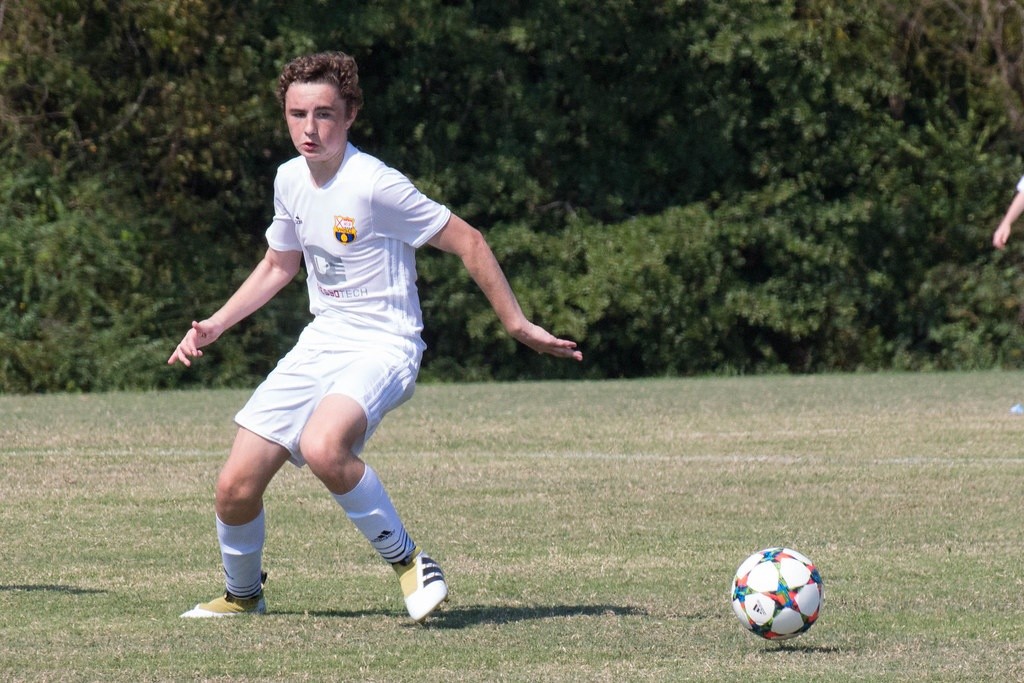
xmin=730 ymin=547 xmax=825 ymax=641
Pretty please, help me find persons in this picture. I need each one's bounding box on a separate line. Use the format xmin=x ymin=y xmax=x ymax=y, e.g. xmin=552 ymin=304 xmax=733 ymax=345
xmin=992 ymin=172 xmax=1024 ymax=252
xmin=167 ymin=50 xmax=583 ymax=623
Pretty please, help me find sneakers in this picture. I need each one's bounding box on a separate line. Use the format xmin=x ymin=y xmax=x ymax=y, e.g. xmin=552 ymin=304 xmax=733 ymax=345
xmin=180 ymin=591 xmax=268 ymax=617
xmin=390 ymin=546 xmax=448 ymax=620
